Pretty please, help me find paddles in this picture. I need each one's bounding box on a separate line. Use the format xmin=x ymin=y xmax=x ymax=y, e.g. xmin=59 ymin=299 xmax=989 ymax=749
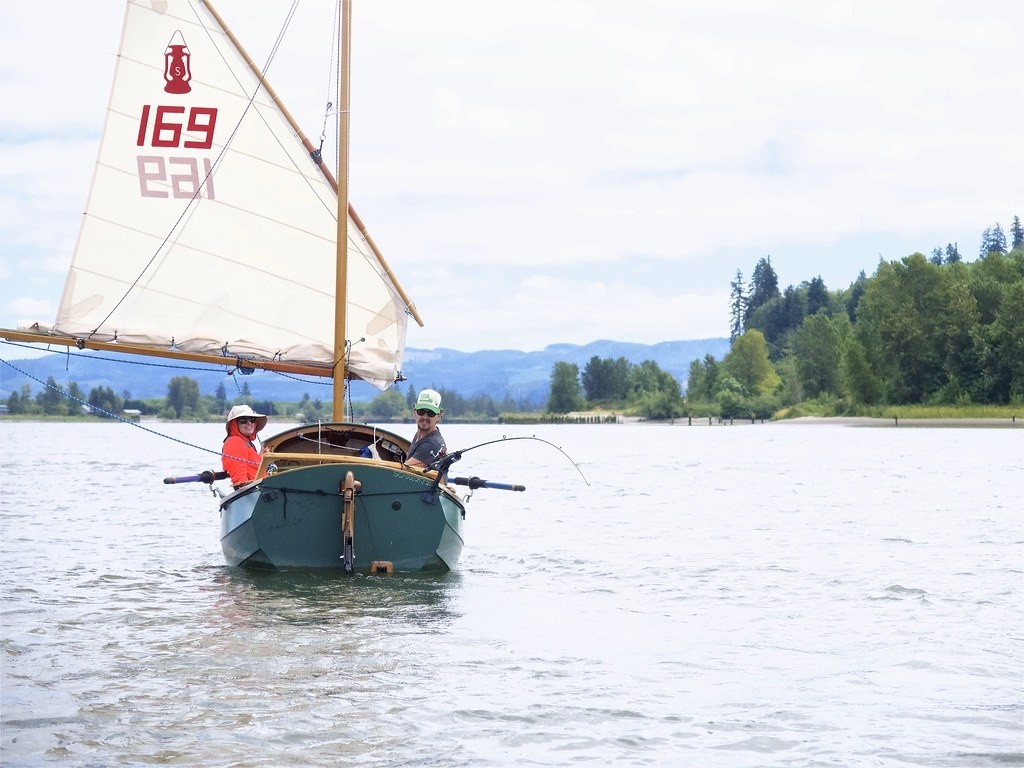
xmin=446 ymin=478 xmax=526 ymax=491
xmin=165 ymin=469 xmax=230 ymax=484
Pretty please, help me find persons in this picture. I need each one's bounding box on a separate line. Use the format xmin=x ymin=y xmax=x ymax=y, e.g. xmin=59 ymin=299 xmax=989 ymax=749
xmin=403 ymin=390 xmax=446 ymax=469
xmin=222 ymin=405 xmax=268 ymax=490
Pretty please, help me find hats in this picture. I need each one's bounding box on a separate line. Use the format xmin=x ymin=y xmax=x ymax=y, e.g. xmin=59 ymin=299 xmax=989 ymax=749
xmin=225 ymin=405 xmax=268 ymax=435
xmin=415 ymin=389 xmax=441 ymax=414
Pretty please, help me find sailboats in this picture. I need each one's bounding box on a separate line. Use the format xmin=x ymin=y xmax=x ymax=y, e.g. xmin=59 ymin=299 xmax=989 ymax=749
xmin=0 ymin=0 xmax=529 ymax=580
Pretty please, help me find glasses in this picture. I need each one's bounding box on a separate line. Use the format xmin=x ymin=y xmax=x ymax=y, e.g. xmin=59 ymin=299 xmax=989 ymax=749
xmin=417 ymin=409 xmax=436 ymax=417
xmin=237 ymin=417 xmax=256 ymax=424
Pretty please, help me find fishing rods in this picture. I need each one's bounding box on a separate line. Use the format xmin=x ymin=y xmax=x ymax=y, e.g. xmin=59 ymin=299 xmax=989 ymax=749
xmin=423 ymin=434 xmax=592 ymax=489
